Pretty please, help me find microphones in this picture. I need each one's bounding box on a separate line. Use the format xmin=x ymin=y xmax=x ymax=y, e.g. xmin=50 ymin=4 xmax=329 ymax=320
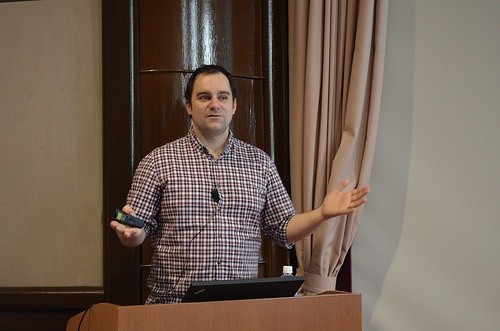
xmin=210 ymin=190 xmax=219 ymax=204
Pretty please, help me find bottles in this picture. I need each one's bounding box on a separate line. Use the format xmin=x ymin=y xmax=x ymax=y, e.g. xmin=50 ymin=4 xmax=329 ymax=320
xmin=279 ymin=265 xmax=294 ymax=278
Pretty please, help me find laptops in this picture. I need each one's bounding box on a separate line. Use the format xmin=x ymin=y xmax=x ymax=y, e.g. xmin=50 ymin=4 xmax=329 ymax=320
xmin=179 ymin=276 xmax=305 ymax=303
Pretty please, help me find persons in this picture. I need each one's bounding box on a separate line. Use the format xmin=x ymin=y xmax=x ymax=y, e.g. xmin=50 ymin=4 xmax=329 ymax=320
xmin=108 ymin=63 xmax=370 ymax=304
